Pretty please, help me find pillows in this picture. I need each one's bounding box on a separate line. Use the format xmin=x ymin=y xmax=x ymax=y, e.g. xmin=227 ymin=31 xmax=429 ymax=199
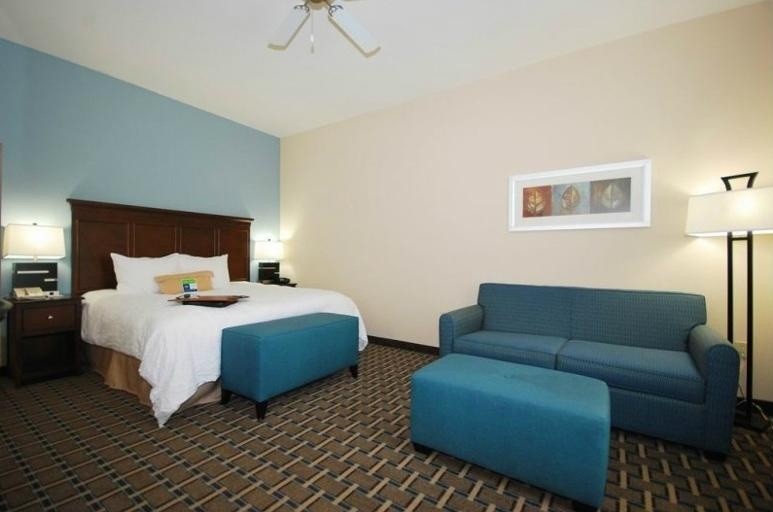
xmin=111 ymin=252 xmax=178 ymax=293
xmin=178 ymin=253 xmax=230 ymax=291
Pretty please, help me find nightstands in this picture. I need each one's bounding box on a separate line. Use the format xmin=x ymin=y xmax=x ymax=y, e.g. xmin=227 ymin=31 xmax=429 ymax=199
xmin=4 ymin=294 xmax=86 ymax=389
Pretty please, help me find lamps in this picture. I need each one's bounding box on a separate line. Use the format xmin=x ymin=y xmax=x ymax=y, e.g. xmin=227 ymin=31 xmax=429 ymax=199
xmin=268 ymin=0 xmax=381 ymax=57
xmin=2 ymin=223 xmax=66 ymax=291
xmin=254 ymin=239 xmax=283 ymax=281
xmin=683 ymin=171 xmax=773 ymax=432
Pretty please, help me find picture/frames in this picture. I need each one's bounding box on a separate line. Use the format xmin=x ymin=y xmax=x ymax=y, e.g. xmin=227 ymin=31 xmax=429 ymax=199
xmin=509 ymin=159 xmax=651 ymax=233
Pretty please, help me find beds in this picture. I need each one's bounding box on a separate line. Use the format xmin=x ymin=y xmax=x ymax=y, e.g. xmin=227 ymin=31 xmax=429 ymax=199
xmin=67 ymin=197 xmax=369 ymax=429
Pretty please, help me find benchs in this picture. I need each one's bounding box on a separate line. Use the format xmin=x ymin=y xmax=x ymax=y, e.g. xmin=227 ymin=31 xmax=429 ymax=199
xmin=222 ymin=312 xmax=357 ymax=421
xmin=410 ymin=353 xmax=611 ymax=511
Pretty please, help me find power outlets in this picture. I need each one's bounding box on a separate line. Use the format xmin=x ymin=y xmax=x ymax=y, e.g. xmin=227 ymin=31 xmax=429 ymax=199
xmin=735 ymin=341 xmax=747 ymax=358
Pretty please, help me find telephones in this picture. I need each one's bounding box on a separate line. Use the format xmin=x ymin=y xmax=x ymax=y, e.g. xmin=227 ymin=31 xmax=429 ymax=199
xmin=13 ymin=287 xmax=44 ymax=300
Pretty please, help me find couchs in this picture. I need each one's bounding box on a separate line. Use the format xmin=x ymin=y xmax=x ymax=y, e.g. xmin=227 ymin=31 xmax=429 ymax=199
xmin=439 ymin=284 xmax=740 ymax=460
